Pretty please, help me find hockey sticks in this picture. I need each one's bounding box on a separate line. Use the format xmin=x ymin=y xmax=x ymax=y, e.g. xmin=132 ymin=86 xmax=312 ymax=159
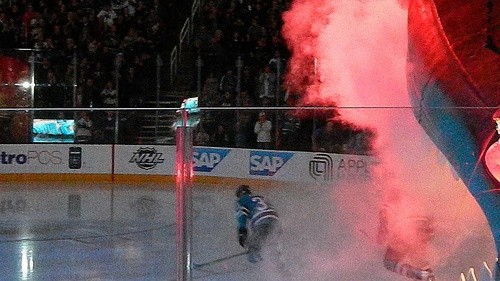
xmin=193 ymin=251 xmax=249 ymax=268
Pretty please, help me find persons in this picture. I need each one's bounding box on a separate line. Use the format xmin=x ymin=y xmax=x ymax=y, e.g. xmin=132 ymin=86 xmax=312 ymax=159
xmin=235 ymin=184 xmax=282 ymax=263
xmin=0 ymin=0 xmax=370 ymax=155
xmin=376 ymin=195 xmax=436 ymax=281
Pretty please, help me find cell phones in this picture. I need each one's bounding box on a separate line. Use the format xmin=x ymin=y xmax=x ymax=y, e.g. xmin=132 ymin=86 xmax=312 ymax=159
xmin=68 ymin=147 xmax=82 ymax=169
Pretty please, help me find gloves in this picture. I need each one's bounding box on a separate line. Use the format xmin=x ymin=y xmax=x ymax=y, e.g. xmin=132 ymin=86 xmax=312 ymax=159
xmin=239 ymin=229 xmax=247 ymax=247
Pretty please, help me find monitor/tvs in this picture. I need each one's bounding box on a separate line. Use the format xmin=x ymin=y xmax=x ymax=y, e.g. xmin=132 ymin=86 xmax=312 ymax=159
xmin=32 ymin=118 xmax=75 ymax=143
xmin=181 ymin=96 xmax=199 ymax=109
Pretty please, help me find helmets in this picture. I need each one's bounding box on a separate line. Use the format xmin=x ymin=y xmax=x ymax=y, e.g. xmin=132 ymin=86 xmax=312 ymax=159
xmin=235 ymin=184 xmax=250 ymax=199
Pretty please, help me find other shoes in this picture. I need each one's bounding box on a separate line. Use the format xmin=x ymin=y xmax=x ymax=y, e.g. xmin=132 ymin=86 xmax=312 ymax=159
xmin=407 ymin=265 xmax=436 ymax=281
